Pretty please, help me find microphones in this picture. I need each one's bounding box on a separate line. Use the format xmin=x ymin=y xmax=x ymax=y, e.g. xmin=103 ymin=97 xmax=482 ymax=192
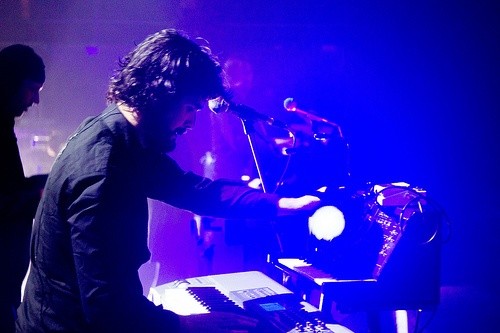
xmin=208 ymin=95 xmax=268 ymax=121
xmin=283 ymin=97 xmax=337 ymax=126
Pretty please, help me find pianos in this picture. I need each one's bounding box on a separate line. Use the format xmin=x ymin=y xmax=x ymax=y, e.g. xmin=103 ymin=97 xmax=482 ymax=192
xmin=146 ymin=268 xmax=356 ymax=333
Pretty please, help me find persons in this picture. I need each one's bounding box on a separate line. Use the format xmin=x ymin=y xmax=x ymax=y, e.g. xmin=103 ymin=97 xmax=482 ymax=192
xmin=1 ymin=45 xmax=50 ymax=333
xmin=17 ymin=28 xmax=347 ymax=333
